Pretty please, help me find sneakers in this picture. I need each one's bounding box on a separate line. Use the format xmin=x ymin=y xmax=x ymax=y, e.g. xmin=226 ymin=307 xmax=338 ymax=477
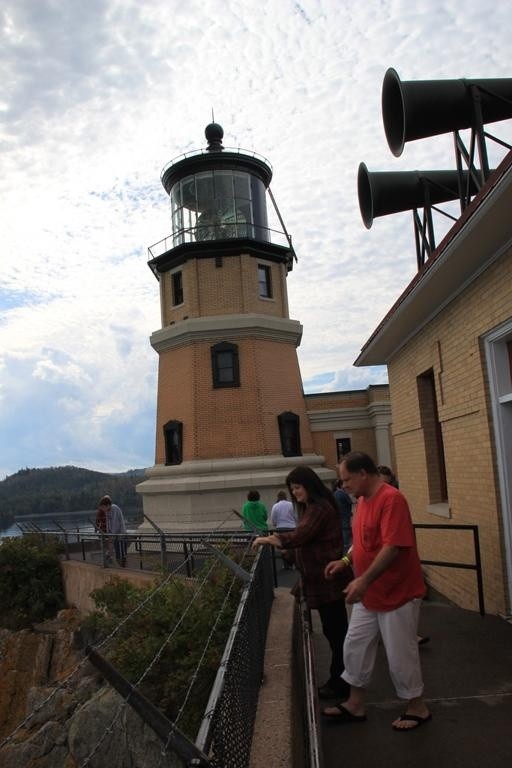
xmin=318 ymin=678 xmax=351 ymax=699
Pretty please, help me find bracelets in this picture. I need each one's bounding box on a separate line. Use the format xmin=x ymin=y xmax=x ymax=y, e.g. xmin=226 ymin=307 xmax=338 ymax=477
xmin=342 ymin=556 xmax=352 ymax=567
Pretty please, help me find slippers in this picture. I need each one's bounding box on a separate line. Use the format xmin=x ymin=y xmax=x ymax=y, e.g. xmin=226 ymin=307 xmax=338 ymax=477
xmin=320 ymin=704 xmax=366 ymax=721
xmin=392 ymin=712 xmax=432 ymax=731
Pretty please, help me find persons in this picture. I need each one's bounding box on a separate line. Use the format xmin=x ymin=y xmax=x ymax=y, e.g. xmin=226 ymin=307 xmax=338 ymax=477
xmin=242 ymin=489 xmax=268 ymax=531
xmin=96 ymin=495 xmax=113 ymax=561
xmin=270 ymin=490 xmax=299 ymax=571
xmin=320 ymin=451 xmax=432 ymax=730
xmin=251 ymin=466 xmax=349 ymax=699
xmin=99 ymin=498 xmax=127 ymax=567
xmin=376 ymin=466 xmax=399 ymax=489
xmin=332 ymin=479 xmax=351 ymax=554
